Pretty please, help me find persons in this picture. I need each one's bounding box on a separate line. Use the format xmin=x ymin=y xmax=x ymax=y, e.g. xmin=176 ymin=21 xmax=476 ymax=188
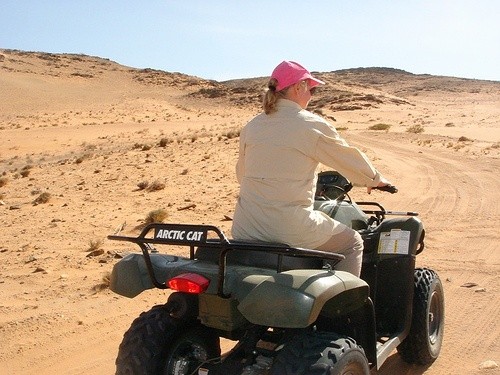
xmin=231 ymin=60 xmax=392 ymax=279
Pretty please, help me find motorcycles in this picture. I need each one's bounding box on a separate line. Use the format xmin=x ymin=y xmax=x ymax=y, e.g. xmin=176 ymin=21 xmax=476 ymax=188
xmin=107 ymin=169 xmax=446 ymax=375
